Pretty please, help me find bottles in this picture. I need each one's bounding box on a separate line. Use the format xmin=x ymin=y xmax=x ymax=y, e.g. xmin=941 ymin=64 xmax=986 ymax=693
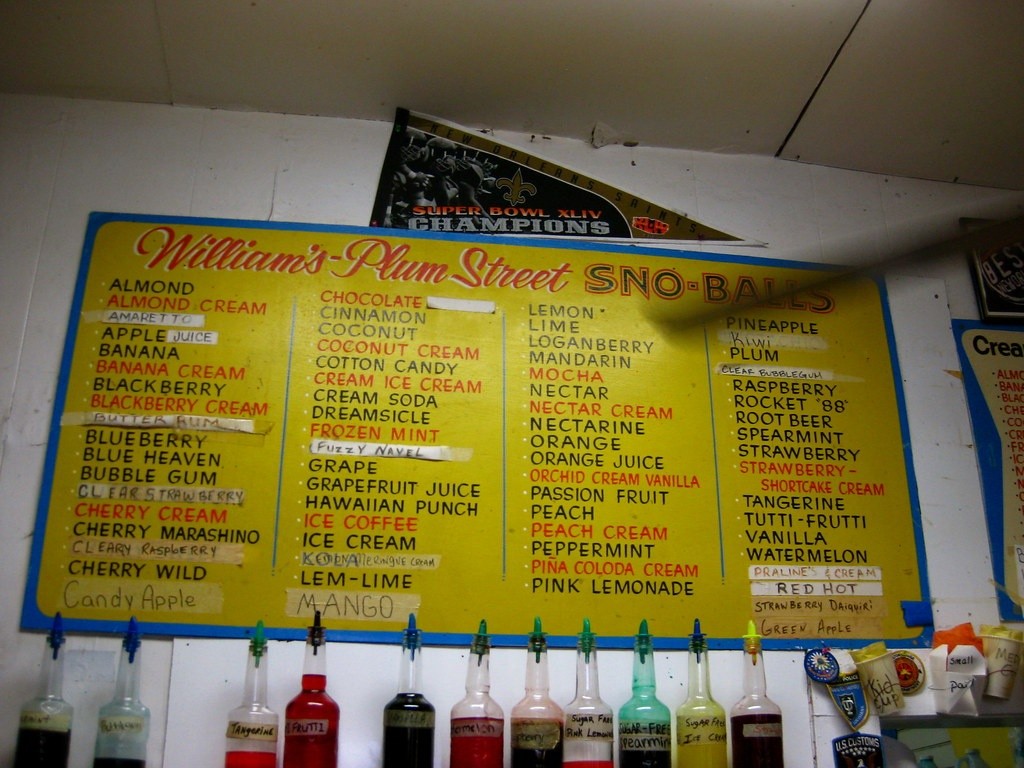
xmin=283 ymin=610 xmax=341 ymax=768
xmin=675 ymin=618 xmax=727 ymax=768
xmin=449 ymin=618 xmax=506 ymax=768
xmin=730 ymin=619 xmax=785 ymax=768
xmin=93 ymin=615 xmax=150 ymax=768
xmin=618 ymin=618 xmax=672 ymax=768
xmin=510 ymin=617 xmax=565 ymax=768
xmin=224 ymin=621 xmax=279 ymax=768
xmin=562 ymin=618 xmax=614 ymax=768
xmin=379 ymin=613 xmax=436 ymax=768
xmin=12 ymin=610 xmax=73 ymax=768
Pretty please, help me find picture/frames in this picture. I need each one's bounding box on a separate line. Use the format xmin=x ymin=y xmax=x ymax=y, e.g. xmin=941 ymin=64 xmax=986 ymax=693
xmin=958 ymin=215 xmax=1024 ymax=324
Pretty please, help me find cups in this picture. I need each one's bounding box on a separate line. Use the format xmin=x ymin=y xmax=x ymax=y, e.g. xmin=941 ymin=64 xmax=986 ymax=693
xmin=975 ymin=635 xmax=1024 ymax=700
xmin=854 ymin=651 xmax=905 ymax=717
